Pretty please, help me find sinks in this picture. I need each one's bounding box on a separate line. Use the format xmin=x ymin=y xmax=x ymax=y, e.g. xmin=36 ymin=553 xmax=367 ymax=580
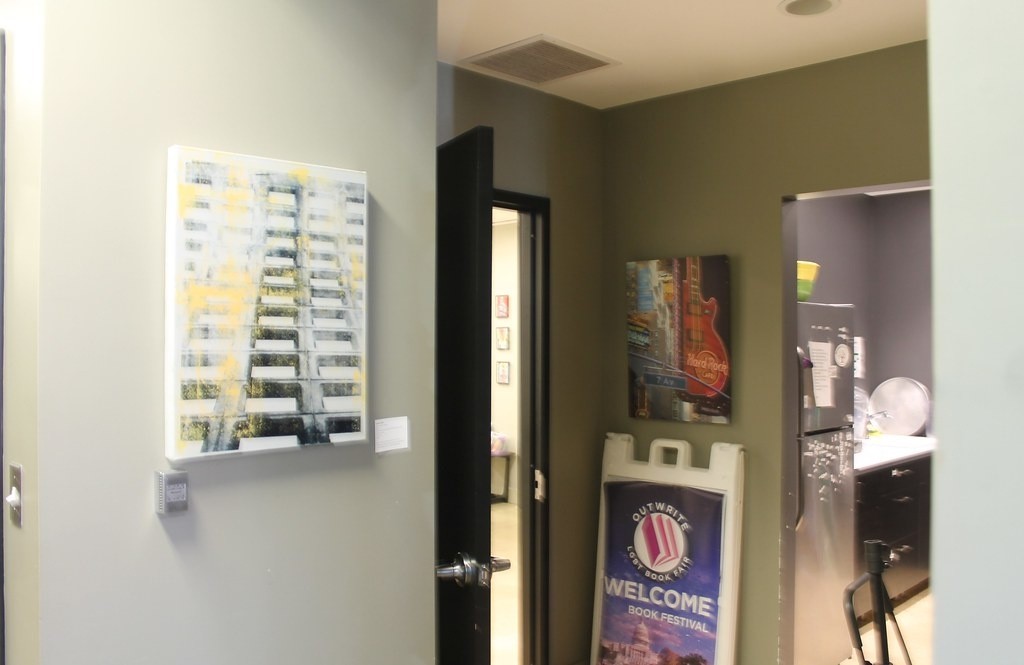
xmin=854 ymin=440 xmax=924 ymax=456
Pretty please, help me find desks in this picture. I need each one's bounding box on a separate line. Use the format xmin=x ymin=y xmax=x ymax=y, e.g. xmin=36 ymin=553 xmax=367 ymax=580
xmin=492 ymin=450 xmax=515 ymax=503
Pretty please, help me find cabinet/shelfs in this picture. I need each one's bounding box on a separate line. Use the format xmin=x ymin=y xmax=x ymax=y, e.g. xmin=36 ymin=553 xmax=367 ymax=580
xmin=853 ymin=456 xmax=933 ymax=631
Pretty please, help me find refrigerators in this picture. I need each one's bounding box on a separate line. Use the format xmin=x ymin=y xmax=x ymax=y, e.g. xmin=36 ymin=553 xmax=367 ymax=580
xmin=794 ymin=302 xmax=854 ymax=665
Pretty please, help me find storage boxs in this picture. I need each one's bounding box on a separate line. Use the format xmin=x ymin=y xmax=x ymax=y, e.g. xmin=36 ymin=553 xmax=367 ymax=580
xmin=491 ymin=430 xmax=506 ymax=452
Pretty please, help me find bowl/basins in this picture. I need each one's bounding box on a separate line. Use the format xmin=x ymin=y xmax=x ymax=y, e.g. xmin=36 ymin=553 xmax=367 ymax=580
xmin=797 ymin=261 xmax=819 ymax=281
xmin=796 ymin=279 xmax=812 ymax=302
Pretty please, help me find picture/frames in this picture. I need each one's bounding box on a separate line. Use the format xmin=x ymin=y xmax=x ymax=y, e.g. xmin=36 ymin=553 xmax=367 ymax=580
xmin=497 ymin=327 xmax=510 ymax=351
xmin=495 ymin=295 xmax=509 ymax=319
xmin=497 ymin=362 xmax=509 ymax=385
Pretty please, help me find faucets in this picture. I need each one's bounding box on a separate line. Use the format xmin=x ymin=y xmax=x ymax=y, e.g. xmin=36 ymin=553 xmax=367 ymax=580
xmin=864 ymin=410 xmax=892 ymax=439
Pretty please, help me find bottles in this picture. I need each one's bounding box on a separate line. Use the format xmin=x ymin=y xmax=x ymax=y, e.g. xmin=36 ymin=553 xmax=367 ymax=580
xmin=671 ymin=394 xmax=679 ymax=420
